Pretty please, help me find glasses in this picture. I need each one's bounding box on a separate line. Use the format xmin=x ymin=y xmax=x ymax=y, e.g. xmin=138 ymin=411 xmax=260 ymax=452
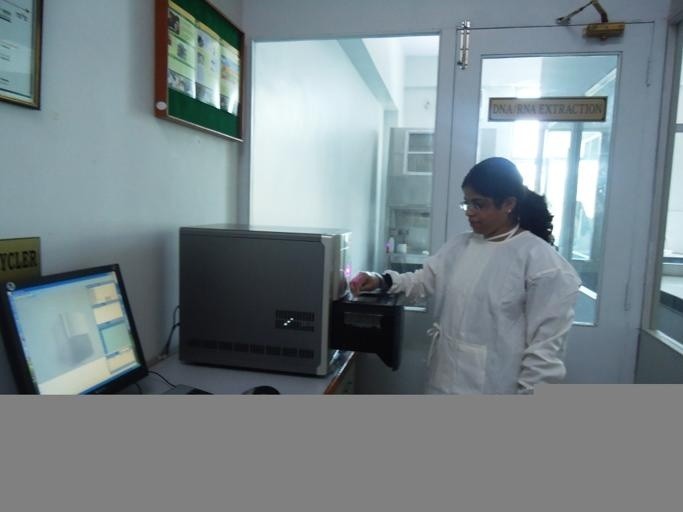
xmin=458 ymin=199 xmax=487 ymax=211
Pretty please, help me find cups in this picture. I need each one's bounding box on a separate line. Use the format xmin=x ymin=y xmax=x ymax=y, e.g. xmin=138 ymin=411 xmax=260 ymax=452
xmin=397 ymin=244 xmax=407 ymax=253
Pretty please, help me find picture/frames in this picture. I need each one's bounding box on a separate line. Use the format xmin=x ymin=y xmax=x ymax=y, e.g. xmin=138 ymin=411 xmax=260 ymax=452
xmin=155 ymin=0 xmax=245 ymax=145
xmin=0 ymin=0 xmax=43 ymax=111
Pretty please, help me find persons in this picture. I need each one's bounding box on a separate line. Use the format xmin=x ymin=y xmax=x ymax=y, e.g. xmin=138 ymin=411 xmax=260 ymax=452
xmin=347 ymin=157 xmax=583 ymax=395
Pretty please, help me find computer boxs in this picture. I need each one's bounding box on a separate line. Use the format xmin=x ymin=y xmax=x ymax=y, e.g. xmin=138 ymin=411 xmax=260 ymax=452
xmin=178 ymin=226 xmax=404 ymax=379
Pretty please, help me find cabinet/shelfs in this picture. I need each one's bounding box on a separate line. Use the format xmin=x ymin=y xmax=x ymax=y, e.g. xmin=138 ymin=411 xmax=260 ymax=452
xmin=384 ymin=126 xmax=434 ymax=270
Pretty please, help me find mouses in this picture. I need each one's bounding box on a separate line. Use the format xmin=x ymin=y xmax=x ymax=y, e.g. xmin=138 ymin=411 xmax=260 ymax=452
xmin=242 ymin=385 xmax=279 ymax=395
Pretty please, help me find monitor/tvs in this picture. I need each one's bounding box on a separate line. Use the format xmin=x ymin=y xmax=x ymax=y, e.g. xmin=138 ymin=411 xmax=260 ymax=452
xmin=0 ymin=263 xmax=149 ymax=395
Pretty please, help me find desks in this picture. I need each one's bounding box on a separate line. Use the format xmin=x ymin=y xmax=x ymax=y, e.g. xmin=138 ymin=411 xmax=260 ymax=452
xmin=111 ymin=347 xmax=362 ymax=395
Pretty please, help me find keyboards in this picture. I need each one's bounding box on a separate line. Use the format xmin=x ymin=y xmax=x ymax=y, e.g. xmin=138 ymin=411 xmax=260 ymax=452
xmin=160 ymin=384 xmax=213 ymax=395
